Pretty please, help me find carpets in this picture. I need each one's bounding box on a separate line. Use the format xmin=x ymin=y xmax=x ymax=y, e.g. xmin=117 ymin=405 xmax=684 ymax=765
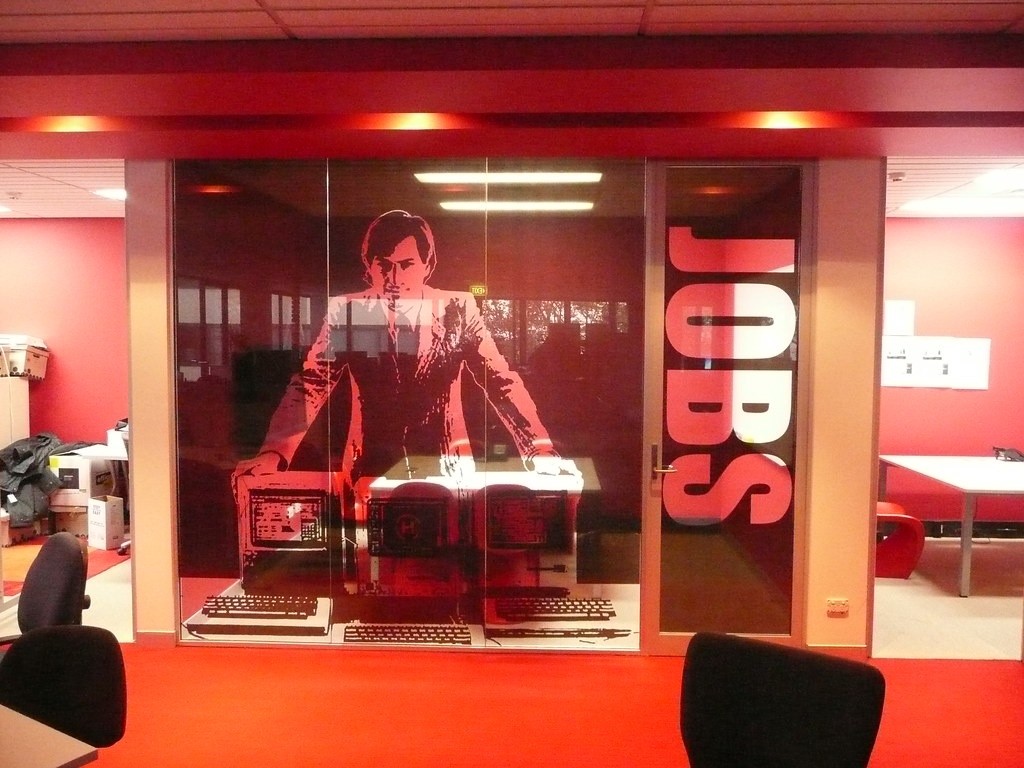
xmin=0 ymin=526 xmax=131 ymax=595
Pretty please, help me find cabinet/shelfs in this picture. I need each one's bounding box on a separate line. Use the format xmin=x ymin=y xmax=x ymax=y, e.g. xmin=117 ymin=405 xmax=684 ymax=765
xmin=0 ymin=377 xmax=30 ymax=451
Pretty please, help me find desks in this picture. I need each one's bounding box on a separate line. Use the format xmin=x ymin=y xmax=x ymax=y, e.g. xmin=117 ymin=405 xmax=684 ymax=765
xmin=83 ymin=456 xmax=129 ymax=498
xmin=0 ymin=705 xmax=99 ymax=768
xmin=371 ymin=456 xmax=602 ymax=574
xmin=879 ymin=455 xmax=1024 ymax=598
xmin=177 ymin=443 xmax=257 ymax=565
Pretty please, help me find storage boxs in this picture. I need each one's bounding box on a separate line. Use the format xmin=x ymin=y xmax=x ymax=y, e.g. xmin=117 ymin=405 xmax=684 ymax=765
xmin=107 ymin=424 xmax=129 ymax=455
xmin=0 ymin=334 xmax=50 ymax=380
xmin=0 ymin=446 xmax=119 ymax=548
xmin=87 ymin=495 xmax=124 ymax=551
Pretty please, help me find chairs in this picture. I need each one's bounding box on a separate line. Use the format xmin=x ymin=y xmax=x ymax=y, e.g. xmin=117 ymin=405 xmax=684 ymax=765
xmin=875 ymin=501 xmax=925 ymax=579
xmin=0 ymin=531 xmax=90 ymax=645
xmin=680 ymin=632 xmax=887 ymax=768
xmin=0 ymin=626 xmax=127 ymax=747
